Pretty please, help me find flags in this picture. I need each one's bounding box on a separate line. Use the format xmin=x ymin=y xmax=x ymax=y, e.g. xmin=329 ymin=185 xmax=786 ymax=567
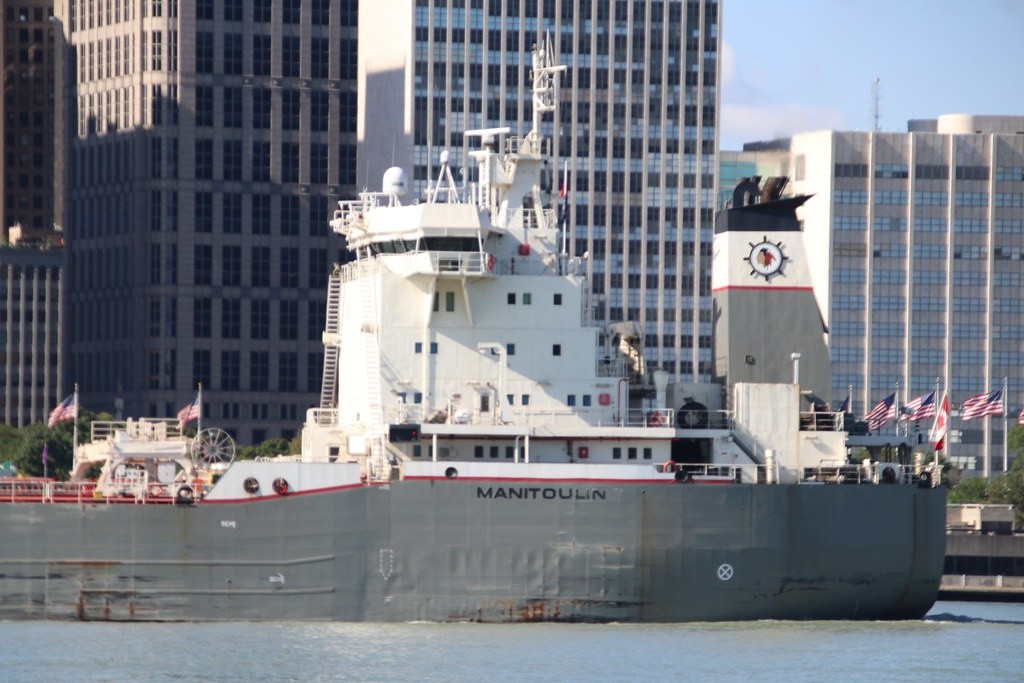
xmin=47 ymin=392 xmax=78 ymax=428
xmin=177 ymin=391 xmax=200 ymax=427
xmin=836 ymin=382 xmax=1024 ymax=451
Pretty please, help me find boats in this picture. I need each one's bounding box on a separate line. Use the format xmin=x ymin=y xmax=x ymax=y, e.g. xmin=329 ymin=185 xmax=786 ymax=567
xmin=0 ymin=28 xmax=961 ymax=624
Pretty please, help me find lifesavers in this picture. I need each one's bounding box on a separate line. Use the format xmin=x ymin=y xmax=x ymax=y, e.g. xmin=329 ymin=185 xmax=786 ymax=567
xmin=663 ymin=460 xmax=675 ymax=473
xmin=677 ymin=402 xmax=709 ymax=429
